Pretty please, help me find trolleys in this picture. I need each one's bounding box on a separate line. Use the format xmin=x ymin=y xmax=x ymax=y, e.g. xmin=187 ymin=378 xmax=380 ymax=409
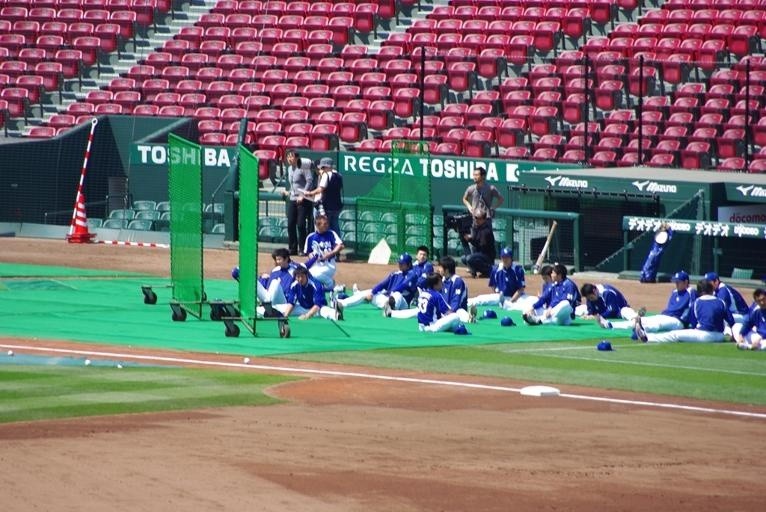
xmin=206 ymin=144 xmax=290 ymax=339
xmin=139 ymin=133 xmax=225 ymax=323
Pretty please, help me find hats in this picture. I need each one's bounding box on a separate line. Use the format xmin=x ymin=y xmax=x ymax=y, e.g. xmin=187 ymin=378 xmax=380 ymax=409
xmin=398 ymin=253 xmax=412 ymax=265
xmin=597 ymin=340 xmax=616 ymax=351
xmin=501 ymin=246 xmax=513 ymax=257
xmin=317 ymin=157 xmax=333 ymax=169
xmin=670 ymin=271 xmax=690 ymax=283
xmin=704 ymin=271 xmax=719 ymax=280
xmin=479 ymin=310 xmax=497 ymax=320
xmin=455 ymin=327 xmax=471 ymax=335
xmin=500 ymin=316 xmax=517 ymax=326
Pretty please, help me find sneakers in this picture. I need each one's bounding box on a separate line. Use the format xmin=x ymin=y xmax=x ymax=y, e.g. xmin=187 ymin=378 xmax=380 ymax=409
xmin=737 ymin=343 xmax=753 ymax=350
xmin=596 ymin=313 xmax=609 ymax=329
xmin=385 ymin=296 xmax=397 ymax=318
xmin=328 ymin=283 xmax=361 ymax=320
xmin=635 ymin=307 xmax=647 ymax=343
xmin=523 ymin=312 xmax=541 ymax=325
xmin=468 ymin=305 xmax=477 ymax=324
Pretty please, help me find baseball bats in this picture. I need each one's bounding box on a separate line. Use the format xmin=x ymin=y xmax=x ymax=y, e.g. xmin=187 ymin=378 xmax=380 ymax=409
xmin=533 ymin=220 xmax=558 ymax=273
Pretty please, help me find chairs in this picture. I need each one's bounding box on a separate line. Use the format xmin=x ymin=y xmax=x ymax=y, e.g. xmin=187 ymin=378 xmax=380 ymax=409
xmin=1 ymin=0 xmax=766 ymax=181
xmin=82 ymin=197 xmax=534 ymax=257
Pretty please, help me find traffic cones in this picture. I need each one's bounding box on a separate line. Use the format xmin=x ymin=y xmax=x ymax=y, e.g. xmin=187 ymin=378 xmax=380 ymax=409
xmin=65 ymin=191 xmax=96 ymax=244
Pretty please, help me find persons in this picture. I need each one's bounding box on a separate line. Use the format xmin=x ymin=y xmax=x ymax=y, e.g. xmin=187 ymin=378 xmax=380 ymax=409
xmin=281 ymin=151 xmax=313 ymax=254
xmin=463 ymin=167 xmax=504 ymax=255
xmin=316 ymin=160 xmax=337 ymax=188
xmin=304 ymin=157 xmax=344 ymax=260
xmin=232 ymin=211 xmax=766 ymax=352
